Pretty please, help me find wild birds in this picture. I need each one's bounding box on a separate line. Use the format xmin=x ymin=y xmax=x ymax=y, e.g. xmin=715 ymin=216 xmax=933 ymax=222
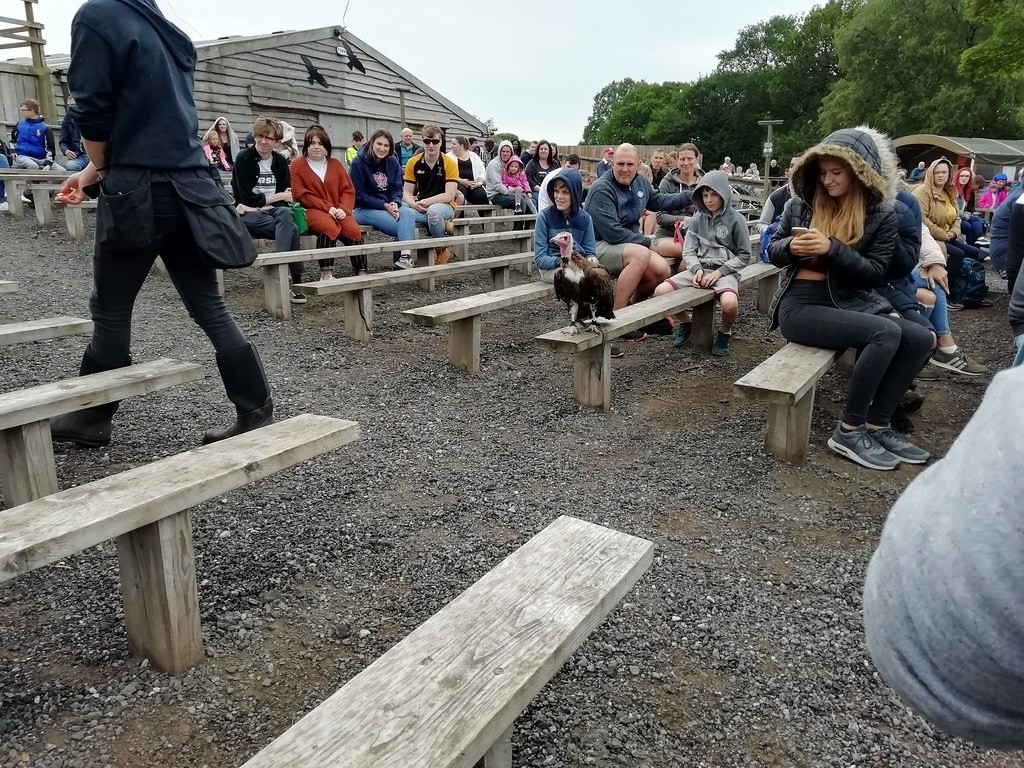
xmin=548 ymin=229 xmax=617 ymax=336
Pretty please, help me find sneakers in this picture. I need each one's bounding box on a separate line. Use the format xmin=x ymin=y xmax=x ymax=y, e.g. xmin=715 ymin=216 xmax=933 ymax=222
xmin=674 ymin=316 xmax=732 ymax=356
xmin=914 ymin=344 xmax=987 ymax=380
xmin=827 ymin=422 xmax=931 ymax=471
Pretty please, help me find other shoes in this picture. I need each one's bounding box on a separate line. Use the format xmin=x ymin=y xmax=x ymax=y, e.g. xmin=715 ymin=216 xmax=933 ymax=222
xmin=394 ymin=255 xmax=415 ymax=269
xmin=290 ymin=286 xmax=308 ymax=304
xmin=515 ymin=205 xmax=523 ymax=212
xmin=320 ymin=275 xmax=337 ymax=281
xmin=946 ymin=301 xmax=964 ymax=311
xmin=891 ymin=393 xmax=925 ymax=433
xmin=434 ymin=219 xmax=454 ymax=266
xmin=974 ymin=237 xmax=990 ymax=245
xmin=289 ymin=275 xmax=293 ymax=284
xmin=0 ymin=202 xmax=9 ymax=211
xmin=393 ymin=267 xmax=403 ymax=271
xmin=4 ymin=192 xmax=32 ymax=202
xmin=983 ymin=256 xmax=991 ymax=261
xmin=23 ymin=192 xmax=35 ymax=209
xmin=620 ymin=330 xmax=649 ymax=342
xmin=611 ymin=344 xmax=624 ymax=357
xmin=979 ymin=298 xmax=993 ymax=306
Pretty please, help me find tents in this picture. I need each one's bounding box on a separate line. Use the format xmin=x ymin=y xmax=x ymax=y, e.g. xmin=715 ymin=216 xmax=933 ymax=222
xmin=890 ymin=134 xmax=1024 ymax=181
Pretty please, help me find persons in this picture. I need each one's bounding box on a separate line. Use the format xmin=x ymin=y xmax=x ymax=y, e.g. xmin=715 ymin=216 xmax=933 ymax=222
xmin=779 ymin=168 xmax=789 ymax=187
xmin=201 ymin=116 xmax=240 ymax=171
xmin=865 ymin=361 xmax=1024 ymax=751
xmin=756 ymin=125 xmax=993 ymax=471
xmin=289 ymin=124 xmax=368 ymax=280
xmin=245 ymin=118 xmax=298 ymax=161
xmin=349 ymin=129 xmax=415 ymax=269
xmin=394 ymin=127 xmax=421 ymax=186
xmin=596 ymin=148 xmax=614 ymax=179
xmin=0 ymin=154 xmax=32 ymax=210
xmin=9 ymin=98 xmax=67 ymax=209
xmin=446 ymin=137 xmax=580 ymax=230
xmin=50 ymin=0 xmax=274 ymax=446
xmin=584 ymin=144 xmax=693 ymax=340
xmin=769 ymin=160 xmax=780 ymax=186
xmin=978 ymin=169 xmax=1024 ymax=365
xmin=403 ymin=123 xmax=464 ymax=265
xmin=231 ymin=114 xmax=308 ymax=304
xmin=535 ymin=168 xmax=624 ymax=357
xmin=653 ymin=170 xmax=751 ymax=355
xmin=639 ymin=149 xmax=678 ymax=234
xmin=656 ymin=143 xmax=723 ymax=311
xmin=719 ymin=157 xmax=759 ymax=178
xmin=59 ymin=96 xmax=99 ymax=213
xmin=346 ymin=131 xmax=364 ymax=166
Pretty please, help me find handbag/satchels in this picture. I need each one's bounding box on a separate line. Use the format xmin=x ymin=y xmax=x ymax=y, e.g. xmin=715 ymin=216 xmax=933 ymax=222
xmin=289 ymin=202 xmax=309 ymax=235
xmin=167 ymin=170 xmax=258 ymax=269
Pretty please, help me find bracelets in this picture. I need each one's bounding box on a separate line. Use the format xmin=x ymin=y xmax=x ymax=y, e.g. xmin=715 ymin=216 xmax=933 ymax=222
xmin=94 ymin=165 xmax=106 ymax=172
xmin=257 ymin=206 xmax=260 ymax=213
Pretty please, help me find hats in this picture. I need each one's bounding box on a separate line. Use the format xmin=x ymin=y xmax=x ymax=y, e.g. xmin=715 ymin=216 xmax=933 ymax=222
xmin=604 ymin=148 xmax=614 ymax=154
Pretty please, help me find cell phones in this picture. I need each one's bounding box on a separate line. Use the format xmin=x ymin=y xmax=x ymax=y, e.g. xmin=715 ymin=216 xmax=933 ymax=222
xmin=792 ymin=227 xmax=807 ymax=239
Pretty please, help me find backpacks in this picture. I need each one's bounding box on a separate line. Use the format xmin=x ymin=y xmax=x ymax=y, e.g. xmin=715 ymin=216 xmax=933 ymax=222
xmin=950 ymin=257 xmax=989 ymax=310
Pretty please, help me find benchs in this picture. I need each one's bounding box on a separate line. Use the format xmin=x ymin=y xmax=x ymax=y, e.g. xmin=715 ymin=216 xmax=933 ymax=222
xmin=0 ymin=168 xmax=756 ymax=375
xmin=0 ymin=357 xmax=206 ymax=510
xmin=975 ymin=207 xmax=996 ymax=224
xmin=0 ymin=413 xmax=362 ymax=678
xmin=733 ymin=341 xmax=858 ymax=465
xmin=240 ymin=513 xmax=656 ymax=768
xmin=535 ymin=260 xmax=781 ymax=412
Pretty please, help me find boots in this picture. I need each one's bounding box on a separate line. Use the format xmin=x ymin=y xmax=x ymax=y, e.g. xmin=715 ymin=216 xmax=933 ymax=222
xmin=51 ymin=343 xmax=132 ymax=447
xmin=204 ymin=342 xmax=274 ymax=445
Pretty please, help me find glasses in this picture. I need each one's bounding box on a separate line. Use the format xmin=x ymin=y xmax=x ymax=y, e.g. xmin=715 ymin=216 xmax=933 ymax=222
xmin=423 ymin=139 xmax=440 ymax=145
xmin=255 ymin=134 xmax=279 ymax=142
